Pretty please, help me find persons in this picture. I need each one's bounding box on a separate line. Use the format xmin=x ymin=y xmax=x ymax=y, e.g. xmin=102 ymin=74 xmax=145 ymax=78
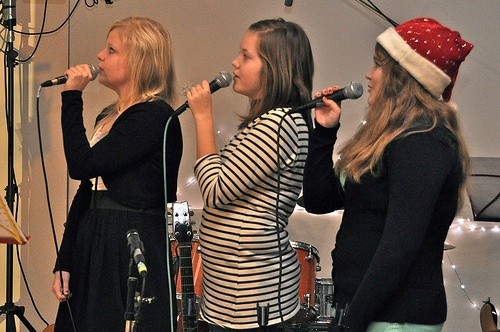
xmin=303 ymin=18 xmax=474 ymax=332
xmin=53 ymin=16 xmax=183 ymax=332
xmin=188 ymin=18 xmax=315 ymax=332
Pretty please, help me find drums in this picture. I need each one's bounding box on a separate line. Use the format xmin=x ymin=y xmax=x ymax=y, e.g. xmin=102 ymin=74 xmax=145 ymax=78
xmin=314 ymin=276 xmax=347 ymax=330
xmin=167 ymin=234 xmax=203 ymax=302
xmin=275 ymin=239 xmax=319 ymax=330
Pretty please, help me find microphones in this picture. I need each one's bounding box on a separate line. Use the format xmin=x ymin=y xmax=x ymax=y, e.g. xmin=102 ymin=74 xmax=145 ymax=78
xmin=41 ymin=63 xmax=98 ymax=87
xmin=126 ymin=228 xmax=148 ymax=274
xmin=171 ymin=70 xmax=233 ymax=119
xmin=286 ymin=81 xmax=363 ymax=116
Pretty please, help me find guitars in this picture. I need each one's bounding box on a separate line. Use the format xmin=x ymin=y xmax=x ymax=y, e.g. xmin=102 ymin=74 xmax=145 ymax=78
xmin=163 ymin=198 xmax=200 ymax=332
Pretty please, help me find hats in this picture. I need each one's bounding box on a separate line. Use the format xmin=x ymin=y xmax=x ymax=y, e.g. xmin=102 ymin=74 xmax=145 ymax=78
xmin=377 ymin=16 xmax=473 ymax=103
